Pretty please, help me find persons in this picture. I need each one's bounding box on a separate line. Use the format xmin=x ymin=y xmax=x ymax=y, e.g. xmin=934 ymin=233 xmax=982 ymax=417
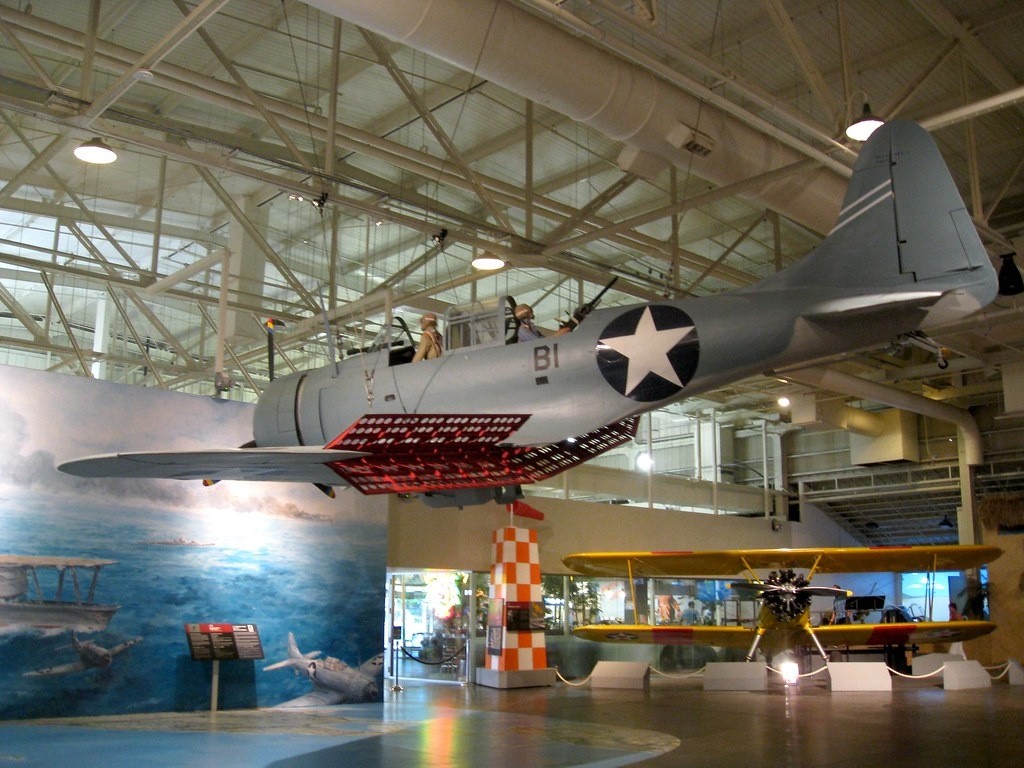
xmin=678 ymin=602 xmax=702 ymax=626
xmin=948 ymin=603 xmax=963 ymax=621
xmin=515 ymin=304 xmax=570 ymax=342
xmin=411 ymin=314 xmax=443 ymax=363
xmin=828 ymin=584 xmax=852 ymax=625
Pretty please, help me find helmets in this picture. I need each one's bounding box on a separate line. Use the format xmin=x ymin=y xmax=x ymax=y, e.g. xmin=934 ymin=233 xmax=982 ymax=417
xmin=514 ymin=303 xmax=533 ymax=320
xmin=420 ymin=313 xmax=436 ymax=331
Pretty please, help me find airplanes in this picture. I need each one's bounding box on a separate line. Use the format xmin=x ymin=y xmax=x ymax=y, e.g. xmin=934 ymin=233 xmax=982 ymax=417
xmin=48 ymin=115 xmax=1002 ymax=508
xmin=558 ymin=543 xmax=998 ymax=678
